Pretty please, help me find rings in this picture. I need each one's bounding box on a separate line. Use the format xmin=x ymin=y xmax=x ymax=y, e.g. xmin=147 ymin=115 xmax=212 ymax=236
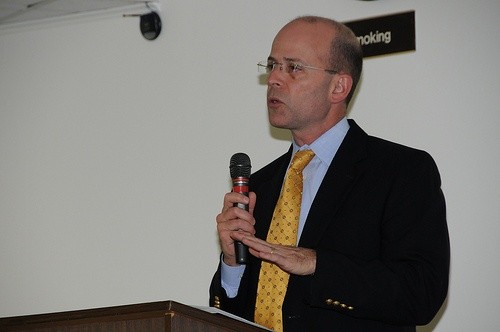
xmin=270 ymin=247 xmax=275 ymax=254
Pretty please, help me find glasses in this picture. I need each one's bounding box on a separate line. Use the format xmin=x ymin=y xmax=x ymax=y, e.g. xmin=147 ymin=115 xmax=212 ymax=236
xmin=257 ymin=60 xmax=341 ymax=74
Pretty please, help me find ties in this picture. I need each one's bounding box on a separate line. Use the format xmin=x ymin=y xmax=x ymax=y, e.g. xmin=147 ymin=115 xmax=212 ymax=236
xmin=254 ymin=150 xmax=315 ymax=332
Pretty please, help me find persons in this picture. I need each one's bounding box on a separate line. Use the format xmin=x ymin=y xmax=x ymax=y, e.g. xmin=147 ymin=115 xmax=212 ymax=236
xmin=208 ymin=16 xmax=450 ymax=332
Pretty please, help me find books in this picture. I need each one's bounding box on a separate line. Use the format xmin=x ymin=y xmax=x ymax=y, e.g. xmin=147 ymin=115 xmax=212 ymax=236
xmin=192 ymin=305 xmax=273 ymax=332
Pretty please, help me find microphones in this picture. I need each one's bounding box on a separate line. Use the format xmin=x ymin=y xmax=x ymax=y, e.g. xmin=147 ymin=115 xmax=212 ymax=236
xmin=229 ymin=153 xmax=252 ymax=264
